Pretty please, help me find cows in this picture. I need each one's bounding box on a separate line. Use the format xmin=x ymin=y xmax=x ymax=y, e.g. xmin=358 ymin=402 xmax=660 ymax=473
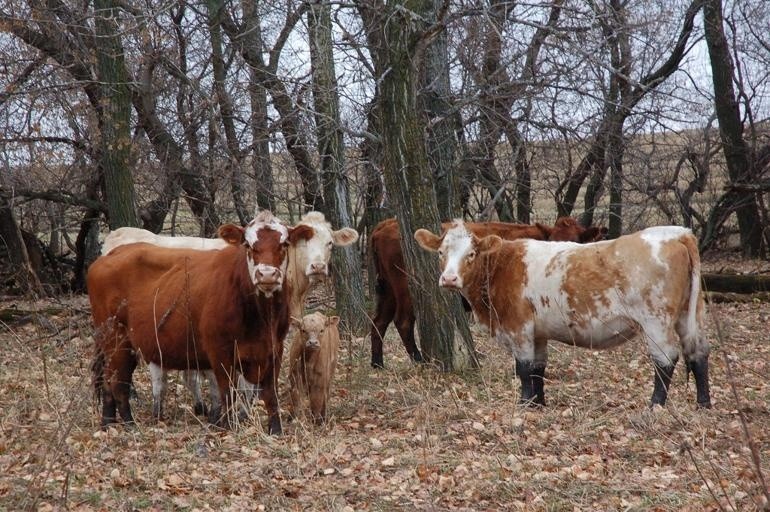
xmin=366 ymin=214 xmax=602 ymax=370
xmin=280 ymin=311 xmax=342 ymax=424
xmin=85 ymin=223 xmax=316 ymax=435
xmin=100 ymin=210 xmax=359 ymax=421
xmin=412 ymin=217 xmax=713 ymax=411
